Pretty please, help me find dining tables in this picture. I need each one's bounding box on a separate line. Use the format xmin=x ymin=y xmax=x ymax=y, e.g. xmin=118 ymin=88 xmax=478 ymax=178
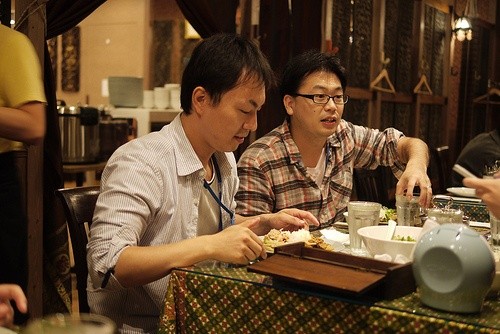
xmin=154 ymin=199 xmax=500 ymax=334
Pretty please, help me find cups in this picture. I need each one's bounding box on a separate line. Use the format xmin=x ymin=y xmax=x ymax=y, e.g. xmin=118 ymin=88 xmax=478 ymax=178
xmin=24 ymin=312 xmax=115 ymax=334
xmin=347 ymin=201 xmax=382 ymax=256
xmin=489 ymin=213 xmax=500 ymax=249
xmin=427 ymin=208 xmax=463 ymax=224
xmin=395 ymin=192 xmax=420 ymax=226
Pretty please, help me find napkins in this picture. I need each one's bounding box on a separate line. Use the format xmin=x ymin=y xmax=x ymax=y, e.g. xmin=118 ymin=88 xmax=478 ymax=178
xmin=319 ymin=228 xmax=350 ymax=245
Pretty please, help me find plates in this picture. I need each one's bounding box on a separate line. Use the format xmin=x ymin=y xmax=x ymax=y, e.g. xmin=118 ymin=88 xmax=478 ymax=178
xmin=447 ymin=187 xmax=476 ymax=198
xmin=107 ymin=76 xmax=143 ymax=107
xmin=258 ymin=235 xmax=274 ymax=254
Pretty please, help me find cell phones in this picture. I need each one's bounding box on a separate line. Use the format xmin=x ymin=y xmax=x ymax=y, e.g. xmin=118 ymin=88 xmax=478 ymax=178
xmin=452 ymin=164 xmax=479 ymax=179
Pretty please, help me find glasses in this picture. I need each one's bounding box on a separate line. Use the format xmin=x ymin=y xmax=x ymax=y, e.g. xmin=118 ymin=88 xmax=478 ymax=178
xmin=293 ymin=93 xmax=349 ymax=104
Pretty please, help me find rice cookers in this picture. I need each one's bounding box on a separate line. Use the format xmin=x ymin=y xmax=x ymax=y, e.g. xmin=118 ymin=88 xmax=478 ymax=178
xmin=58 ymin=100 xmax=100 ymax=163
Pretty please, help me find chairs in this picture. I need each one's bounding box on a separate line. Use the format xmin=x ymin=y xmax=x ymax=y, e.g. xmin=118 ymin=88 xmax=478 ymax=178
xmin=57 ymin=186 xmax=100 ymax=316
xmin=432 ymin=145 xmax=451 ymax=195
xmin=353 ymin=165 xmax=388 ymax=207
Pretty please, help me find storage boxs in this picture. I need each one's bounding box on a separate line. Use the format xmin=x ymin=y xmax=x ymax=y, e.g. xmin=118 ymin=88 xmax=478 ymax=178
xmin=246 ymin=241 xmax=416 ymax=305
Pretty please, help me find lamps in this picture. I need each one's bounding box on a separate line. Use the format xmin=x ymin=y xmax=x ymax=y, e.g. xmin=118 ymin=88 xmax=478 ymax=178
xmin=451 ymin=17 xmax=473 ymax=42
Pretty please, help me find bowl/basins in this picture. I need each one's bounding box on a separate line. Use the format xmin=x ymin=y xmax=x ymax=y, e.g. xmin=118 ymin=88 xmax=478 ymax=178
xmin=343 ymin=212 xmax=348 ymax=222
xmin=142 ymin=83 xmax=181 ymax=110
xmin=356 ymin=225 xmax=421 ymax=260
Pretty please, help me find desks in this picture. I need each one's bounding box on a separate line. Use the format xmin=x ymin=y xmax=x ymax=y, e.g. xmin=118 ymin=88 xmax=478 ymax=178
xmin=112 ymin=108 xmax=179 ymax=137
xmin=62 ymin=162 xmax=106 ymax=188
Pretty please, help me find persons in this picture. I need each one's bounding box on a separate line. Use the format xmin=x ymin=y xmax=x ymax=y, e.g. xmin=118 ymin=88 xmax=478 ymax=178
xmin=236 ymin=50 xmax=432 ymax=233
xmin=84 ymin=33 xmax=319 ymax=334
xmin=0 ymin=22 xmax=48 ymax=334
xmin=452 ymin=127 xmax=500 ymax=231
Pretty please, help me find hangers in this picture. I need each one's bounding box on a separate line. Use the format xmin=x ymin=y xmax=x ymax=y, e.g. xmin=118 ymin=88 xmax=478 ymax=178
xmin=474 ymin=87 xmax=500 ymax=104
xmin=413 ymin=68 xmax=432 ymax=95
xmin=369 ymin=60 xmax=395 ymax=93
